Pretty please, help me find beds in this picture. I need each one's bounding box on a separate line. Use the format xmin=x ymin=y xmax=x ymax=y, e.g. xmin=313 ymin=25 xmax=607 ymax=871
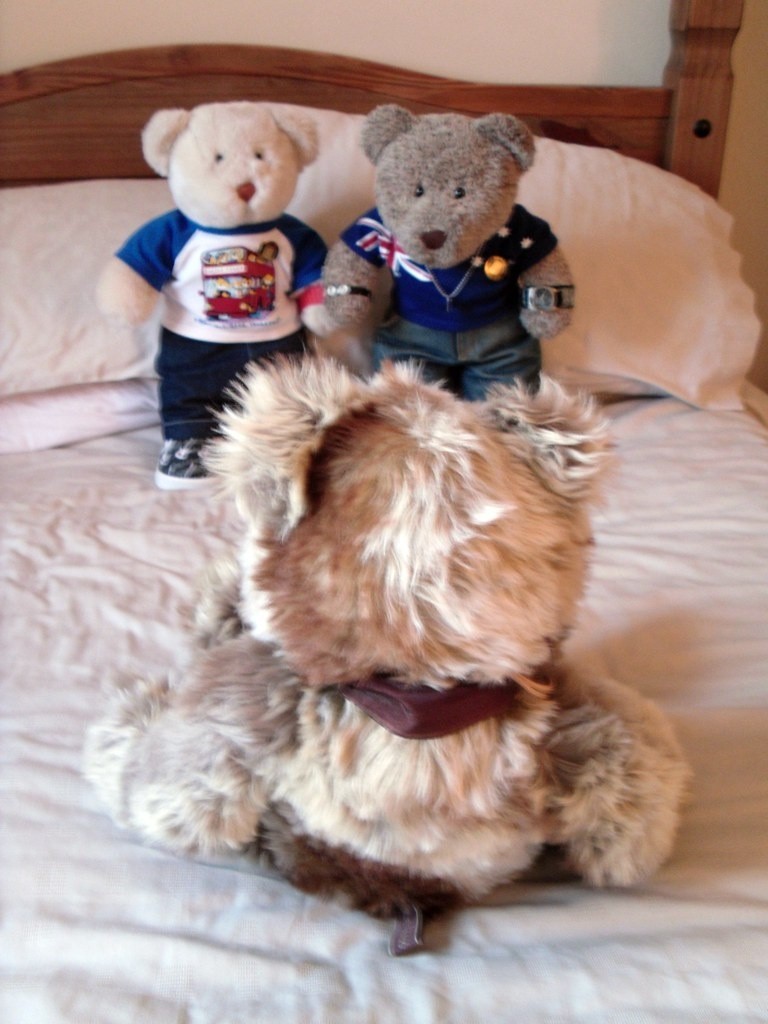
xmin=0 ymin=0 xmax=768 ymax=1024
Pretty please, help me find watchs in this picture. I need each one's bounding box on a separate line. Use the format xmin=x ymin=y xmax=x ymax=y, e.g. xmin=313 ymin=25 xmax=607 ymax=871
xmin=521 ymin=286 xmax=576 ymax=311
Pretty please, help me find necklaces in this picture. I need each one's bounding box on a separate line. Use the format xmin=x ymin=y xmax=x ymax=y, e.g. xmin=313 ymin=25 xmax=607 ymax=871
xmin=425 ymin=243 xmax=482 ymax=312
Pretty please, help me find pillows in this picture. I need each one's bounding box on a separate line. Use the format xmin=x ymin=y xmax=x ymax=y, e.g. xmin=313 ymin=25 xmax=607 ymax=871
xmin=288 ymin=85 xmax=763 ymax=415
xmin=0 ymin=166 xmax=174 ymax=399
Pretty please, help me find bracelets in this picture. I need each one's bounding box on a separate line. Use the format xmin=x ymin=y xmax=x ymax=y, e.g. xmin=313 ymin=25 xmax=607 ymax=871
xmin=323 ymin=285 xmax=372 ymax=297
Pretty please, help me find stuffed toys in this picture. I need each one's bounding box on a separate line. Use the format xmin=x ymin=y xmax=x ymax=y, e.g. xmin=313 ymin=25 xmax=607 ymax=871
xmin=73 ymin=349 xmax=691 ymax=954
xmin=319 ymin=102 xmax=574 ymax=428
xmin=97 ymin=98 xmax=330 ymax=486
